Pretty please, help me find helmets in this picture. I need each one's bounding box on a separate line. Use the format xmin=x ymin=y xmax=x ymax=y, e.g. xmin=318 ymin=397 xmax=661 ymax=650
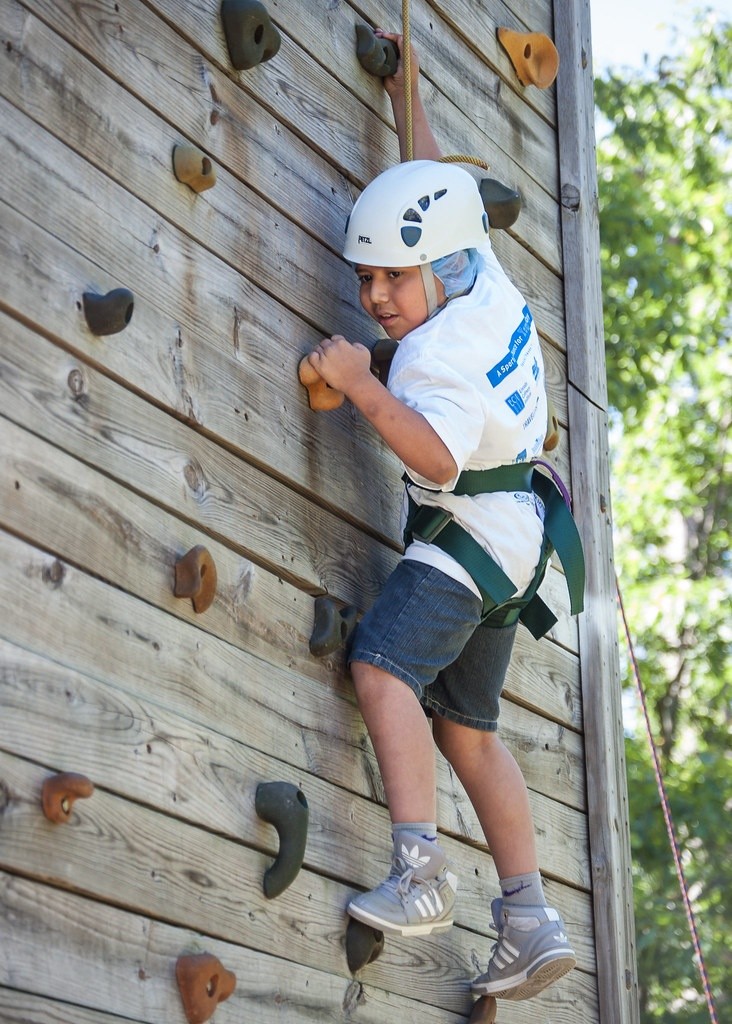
xmin=342 ymin=160 xmax=492 ymax=267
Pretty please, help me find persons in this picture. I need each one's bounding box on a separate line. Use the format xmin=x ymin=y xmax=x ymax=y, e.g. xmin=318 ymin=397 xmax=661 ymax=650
xmin=308 ymin=31 xmax=576 ymax=1001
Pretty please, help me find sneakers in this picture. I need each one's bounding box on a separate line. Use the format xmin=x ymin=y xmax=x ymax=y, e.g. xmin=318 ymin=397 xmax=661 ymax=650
xmin=347 ymin=830 xmax=459 ymax=937
xmin=470 ymin=898 xmax=577 ymax=1001
